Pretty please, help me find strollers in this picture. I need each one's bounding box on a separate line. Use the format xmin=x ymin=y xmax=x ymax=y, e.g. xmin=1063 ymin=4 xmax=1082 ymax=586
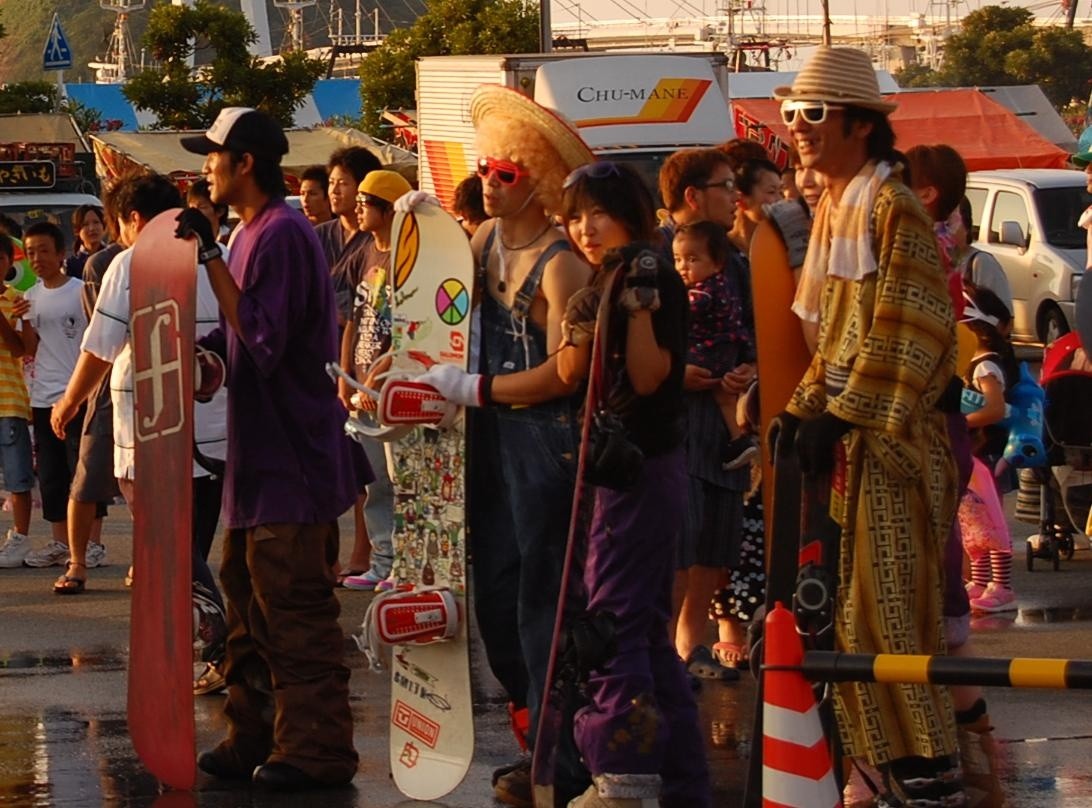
xmin=1024 ymin=331 xmax=1092 ymax=574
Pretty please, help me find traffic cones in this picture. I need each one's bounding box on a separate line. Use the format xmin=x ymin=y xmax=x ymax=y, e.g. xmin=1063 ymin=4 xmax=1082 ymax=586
xmin=760 ymin=599 xmax=845 ymax=808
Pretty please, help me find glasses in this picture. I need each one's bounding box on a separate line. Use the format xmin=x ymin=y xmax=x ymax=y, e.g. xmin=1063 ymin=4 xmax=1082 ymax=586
xmin=562 ymin=161 xmax=620 ymax=189
xmin=356 ymin=193 xmax=372 ymax=202
xmin=477 ymin=157 xmax=530 ymax=187
xmin=684 ymin=180 xmax=736 ymax=193
xmin=780 ymin=101 xmax=850 ymax=126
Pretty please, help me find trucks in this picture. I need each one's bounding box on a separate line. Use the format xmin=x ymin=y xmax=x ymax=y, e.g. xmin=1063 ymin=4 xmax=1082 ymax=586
xmin=413 ymin=49 xmax=740 ymax=238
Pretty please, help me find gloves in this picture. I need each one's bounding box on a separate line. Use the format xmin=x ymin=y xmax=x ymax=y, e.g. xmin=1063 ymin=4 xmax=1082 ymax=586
xmin=392 ymin=190 xmax=441 ymax=214
xmin=413 ymin=364 xmax=487 ymax=406
xmin=173 ymin=207 xmax=222 ymax=264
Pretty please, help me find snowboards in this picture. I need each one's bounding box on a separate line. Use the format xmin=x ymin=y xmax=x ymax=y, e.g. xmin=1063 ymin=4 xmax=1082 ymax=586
xmin=389 ymin=201 xmax=474 ymax=802
xmin=531 ymin=259 xmax=601 ymax=808
xmin=750 ymin=208 xmax=815 ymax=577
xmin=130 ymin=207 xmax=197 ymax=793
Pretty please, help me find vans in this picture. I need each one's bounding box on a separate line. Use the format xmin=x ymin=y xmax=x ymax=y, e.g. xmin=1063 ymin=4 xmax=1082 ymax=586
xmin=963 ymin=168 xmax=1092 ymax=348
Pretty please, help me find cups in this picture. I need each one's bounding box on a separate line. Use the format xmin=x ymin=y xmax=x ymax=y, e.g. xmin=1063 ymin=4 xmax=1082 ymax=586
xmin=21 ymin=300 xmax=35 ymax=320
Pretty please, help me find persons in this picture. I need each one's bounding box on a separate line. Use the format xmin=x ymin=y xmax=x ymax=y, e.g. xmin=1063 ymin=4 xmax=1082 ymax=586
xmin=1 ymin=45 xmax=1091 ymax=808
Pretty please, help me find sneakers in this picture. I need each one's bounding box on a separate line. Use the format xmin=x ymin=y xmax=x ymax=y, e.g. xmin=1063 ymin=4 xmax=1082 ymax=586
xmin=65 ymin=541 xmax=107 ymax=567
xmin=0 ymin=530 xmax=33 ymax=569
xmin=23 ymin=540 xmax=70 ymax=568
xmin=964 ymin=582 xmax=1018 ymax=612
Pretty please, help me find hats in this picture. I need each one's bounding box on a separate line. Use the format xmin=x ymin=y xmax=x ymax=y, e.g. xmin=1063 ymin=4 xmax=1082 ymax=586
xmin=470 ymin=83 xmax=596 ymax=172
xmin=357 ymin=171 xmax=413 ymax=204
xmin=181 ymin=107 xmax=290 ymax=156
xmin=773 ymin=45 xmax=899 ymax=115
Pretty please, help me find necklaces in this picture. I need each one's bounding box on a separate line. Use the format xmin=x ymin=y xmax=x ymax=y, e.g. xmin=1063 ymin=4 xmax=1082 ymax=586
xmin=501 ymin=223 xmax=552 ymax=251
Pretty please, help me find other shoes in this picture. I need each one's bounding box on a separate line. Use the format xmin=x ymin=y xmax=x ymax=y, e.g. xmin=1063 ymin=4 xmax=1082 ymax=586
xmin=566 ymin=784 xmax=644 ymax=808
xmin=495 ymin=766 xmax=584 ymax=807
xmin=376 ymin=575 xmax=397 ymax=593
xmin=343 ymin=575 xmax=378 ymax=590
xmin=195 ymin=746 xmax=260 ymax=778
xmin=253 ymin=759 xmax=354 ymax=786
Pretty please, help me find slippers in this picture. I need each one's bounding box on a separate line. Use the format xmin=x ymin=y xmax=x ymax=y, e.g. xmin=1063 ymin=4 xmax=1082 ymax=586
xmin=685 ymin=639 xmax=753 ymax=684
xmin=52 ymin=575 xmax=86 ymax=593
xmin=334 ymin=568 xmax=367 ymax=586
xmin=194 ymin=661 xmax=227 ymax=695
